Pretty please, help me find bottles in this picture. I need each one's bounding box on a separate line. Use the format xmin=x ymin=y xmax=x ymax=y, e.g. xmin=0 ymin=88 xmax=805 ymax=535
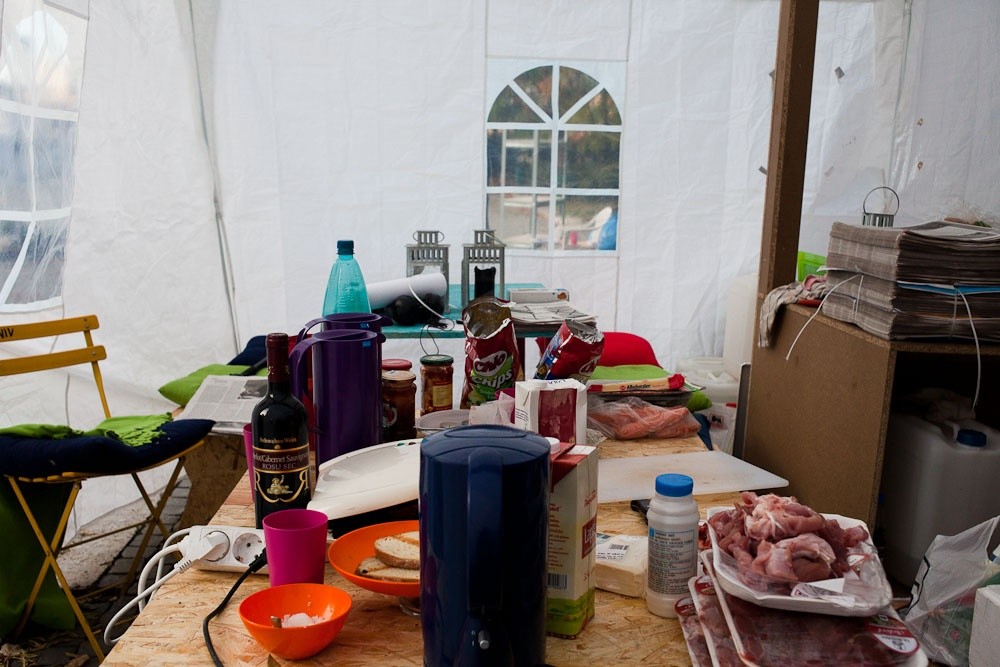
xmin=252 ymin=333 xmax=311 ymax=530
xmin=321 ymin=239 xmax=372 ymax=332
xmin=646 ymin=472 xmax=701 ymax=620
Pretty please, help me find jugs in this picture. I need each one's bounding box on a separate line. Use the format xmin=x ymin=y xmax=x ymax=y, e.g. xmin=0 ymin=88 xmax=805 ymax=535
xmin=289 ymin=329 xmax=386 ymax=482
xmin=296 ymin=311 xmax=385 ymax=445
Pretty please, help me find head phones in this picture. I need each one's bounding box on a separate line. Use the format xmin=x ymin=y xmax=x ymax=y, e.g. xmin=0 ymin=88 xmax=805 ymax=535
xmin=376 ymin=293 xmax=444 ymax=326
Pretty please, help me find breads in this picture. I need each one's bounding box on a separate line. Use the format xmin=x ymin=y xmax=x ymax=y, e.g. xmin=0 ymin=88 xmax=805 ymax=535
xmin=355 ymin=529 xmax=421 ymax=581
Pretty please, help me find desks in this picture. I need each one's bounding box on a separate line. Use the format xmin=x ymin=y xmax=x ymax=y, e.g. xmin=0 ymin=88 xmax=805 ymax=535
xmin=96 ymin=394 xmax=750 ymax=667
xmin=360 ymin=283 xmax=578 ymax=381
xmin=740 ymin=288 xmax=1000 ymax=612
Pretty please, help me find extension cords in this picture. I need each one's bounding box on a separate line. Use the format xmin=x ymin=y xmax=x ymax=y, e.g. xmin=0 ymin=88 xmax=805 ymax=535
xmin=177 ymin=526 xmax=270 ymax=574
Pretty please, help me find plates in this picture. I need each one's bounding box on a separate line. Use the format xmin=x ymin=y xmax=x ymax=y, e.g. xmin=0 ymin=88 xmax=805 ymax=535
xmin=675 ymin=506 xmax=929 ymax=667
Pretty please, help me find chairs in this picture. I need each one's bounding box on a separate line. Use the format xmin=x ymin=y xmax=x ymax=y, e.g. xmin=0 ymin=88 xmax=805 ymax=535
xmin=0 ymin=314 xmax=205 ymax=662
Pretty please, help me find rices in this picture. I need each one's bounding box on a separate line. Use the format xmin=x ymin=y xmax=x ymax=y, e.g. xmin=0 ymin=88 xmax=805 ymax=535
xmin=281 ymin=613 xmax=330 ymax=628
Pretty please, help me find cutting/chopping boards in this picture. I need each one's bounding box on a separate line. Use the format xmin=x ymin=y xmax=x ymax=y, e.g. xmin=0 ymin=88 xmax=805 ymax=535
xmin=596 ymin=451 xmax=789 ymax=504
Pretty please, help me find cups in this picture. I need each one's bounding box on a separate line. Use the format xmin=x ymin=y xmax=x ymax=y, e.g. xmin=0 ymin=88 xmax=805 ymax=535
xmin=418 ymin=423 xmax=552 ymax=667
xmin=242 ymin=422 xmax=254 ymax=503
xmin=262 ymin=510 xmax=328 ymax=588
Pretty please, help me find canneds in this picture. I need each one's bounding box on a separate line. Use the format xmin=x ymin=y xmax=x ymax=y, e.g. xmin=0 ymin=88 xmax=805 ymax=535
xmin=380 ymin=359 xmax=418 ymax=442
xmin=419 ymin=353 xmax=453 ymax=417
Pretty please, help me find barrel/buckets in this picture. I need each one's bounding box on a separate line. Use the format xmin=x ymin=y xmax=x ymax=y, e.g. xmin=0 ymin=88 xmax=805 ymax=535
xmin=873 ymin=415 xmax=1000 ymax=598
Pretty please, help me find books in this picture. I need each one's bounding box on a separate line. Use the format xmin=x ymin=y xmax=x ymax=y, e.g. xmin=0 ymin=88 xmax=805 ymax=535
xmin=172 ymin=375 xmax=269 ymax=437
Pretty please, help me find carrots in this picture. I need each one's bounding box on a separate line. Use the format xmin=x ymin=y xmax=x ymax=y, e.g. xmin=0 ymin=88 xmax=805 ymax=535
xmin=589 ymin=404 xmax=702 ymax=440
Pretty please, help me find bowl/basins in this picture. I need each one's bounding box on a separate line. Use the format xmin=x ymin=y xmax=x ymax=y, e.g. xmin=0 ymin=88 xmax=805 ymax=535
xmin=238 ymin=583 xmax=352 ymax=660
xmin=328 ymin=520 xmax=420 ymax=597
